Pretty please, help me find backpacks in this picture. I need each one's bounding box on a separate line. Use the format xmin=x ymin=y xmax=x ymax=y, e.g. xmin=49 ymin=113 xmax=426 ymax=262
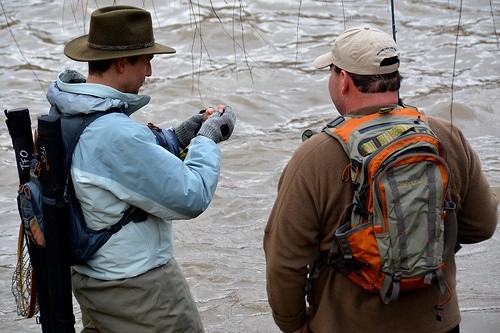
xmin=322 ymin=107 xmax=455 ymax=322
xmin=15 ymin=105 xmax=148 ymax=266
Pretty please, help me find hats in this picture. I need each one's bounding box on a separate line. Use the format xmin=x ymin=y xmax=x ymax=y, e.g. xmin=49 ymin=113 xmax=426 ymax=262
xmin=312 ymin=26 xmax=401 ymax=75
xmin=64 ymin=5 xmax=176 ymax=61
